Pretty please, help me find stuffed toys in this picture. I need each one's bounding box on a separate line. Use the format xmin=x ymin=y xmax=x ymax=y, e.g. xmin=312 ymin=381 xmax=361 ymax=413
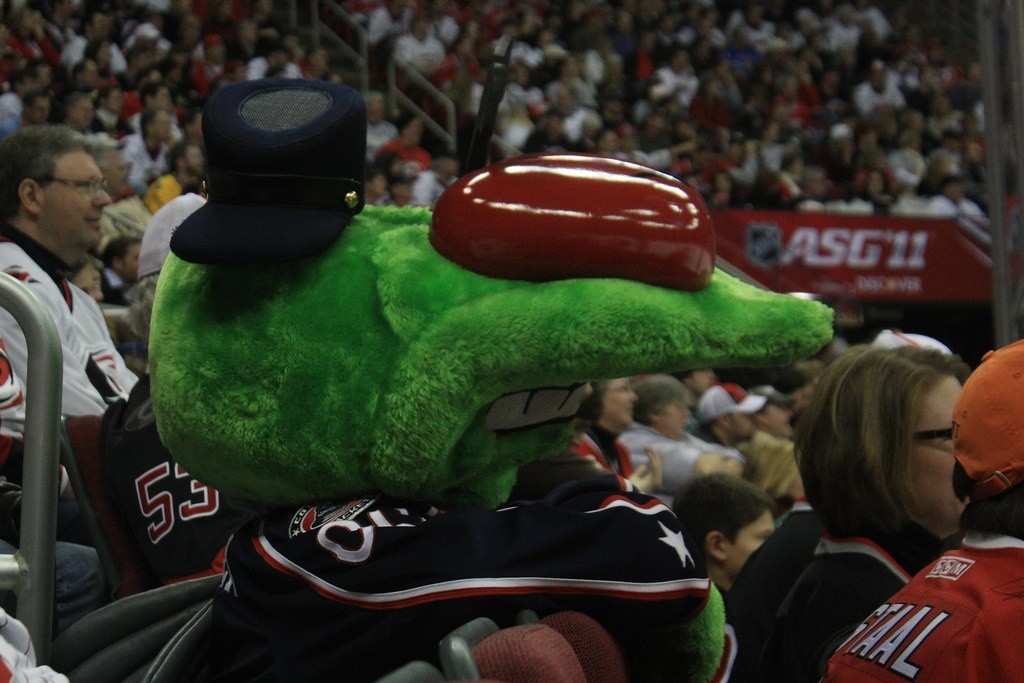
xmin=149 ymin=79 xmax=834 ymax=683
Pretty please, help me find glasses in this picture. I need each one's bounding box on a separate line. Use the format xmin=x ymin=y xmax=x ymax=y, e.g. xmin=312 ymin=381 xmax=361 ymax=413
xmin=53 ymin=174 xmax=112 ymax=198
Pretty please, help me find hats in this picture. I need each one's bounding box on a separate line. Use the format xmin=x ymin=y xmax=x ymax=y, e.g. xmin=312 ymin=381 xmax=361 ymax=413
xmin=136 ymin=192 xmax=207 ymax=279
xmin=951 ymin=338 xmax=1024 ymax=500
xmin=143 ymin=173 xmax=182 ymax=216
xmin=873 ymin=328 xmax=953 ymax=358
xmin=169 ymin=77 xmax=369 ymax=267
xmin=697 ymin=381 xmax=767 ymax=426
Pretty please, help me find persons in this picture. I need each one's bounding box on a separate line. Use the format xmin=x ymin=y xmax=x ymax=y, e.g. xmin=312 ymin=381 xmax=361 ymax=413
xmin=821 ymin=339 xmax=1024 ymax=683
xmin=0 ymin=338 xmax=107 ymax=640
xmin=0 ymin=123 xmax=139 ymax=417
xmin=756 ymin=344 xmax=968 ymax=683
xmin=672 ymin=473 xmax=774 ymax=596
xmin=615 ymin=373 xmax=747 ymax=494
xmin=1 ymin=0 xmax=987 ymax=510
xmin=725 ymin=500 xmax=824 ymax=683
